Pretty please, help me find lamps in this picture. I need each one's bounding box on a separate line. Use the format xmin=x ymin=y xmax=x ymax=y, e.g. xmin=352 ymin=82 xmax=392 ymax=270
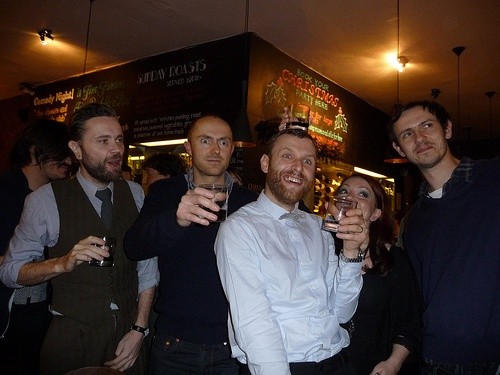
xmin=383 ymin=0 xmax=409 ymax=163
xmin=38 ymin=29 xmax=53 ymax=46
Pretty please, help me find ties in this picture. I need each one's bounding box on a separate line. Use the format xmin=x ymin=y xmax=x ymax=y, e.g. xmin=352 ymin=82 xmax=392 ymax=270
xmin=95 ymin=188 xmax=114 ymax=233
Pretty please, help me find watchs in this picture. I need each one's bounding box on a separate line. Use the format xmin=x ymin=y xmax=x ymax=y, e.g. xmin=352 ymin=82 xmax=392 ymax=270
xmin=130 ymin=324 xmax=150 ymax=337
xmin=339 ymin=248 xmax=362 ymax=262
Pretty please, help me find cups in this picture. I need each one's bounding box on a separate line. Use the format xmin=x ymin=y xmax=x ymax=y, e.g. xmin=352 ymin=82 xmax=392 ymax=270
xmin=321 ymin=196 xmax=358 ymax=233
xmin=88 ymin=235 xmax=117 ymax=267
xmin=198 ymin=183 xmax=228 ymax=223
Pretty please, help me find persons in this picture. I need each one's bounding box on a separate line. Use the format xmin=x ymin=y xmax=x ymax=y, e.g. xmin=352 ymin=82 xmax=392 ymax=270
xmin=124 ymin=115 xmax=259 ymax=375
xmin=214 ymin=128 xmax=369 ymax=375
xmin=0 ymin=119 xmax=245 ymax=375
xmin=0 ymin=103 xmax=160 ymax=375
xmin=279 ymin=104 xmax=422 ymax=375
xmin=391 ymin=208 xmax=405 ymax=249
xmin=389 ymin=99 xmax=500 ymax=375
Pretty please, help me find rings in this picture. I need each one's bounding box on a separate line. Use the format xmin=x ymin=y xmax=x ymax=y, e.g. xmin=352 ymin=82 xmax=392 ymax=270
xmin=359 ymin=226 xmax=363 ymax=233
